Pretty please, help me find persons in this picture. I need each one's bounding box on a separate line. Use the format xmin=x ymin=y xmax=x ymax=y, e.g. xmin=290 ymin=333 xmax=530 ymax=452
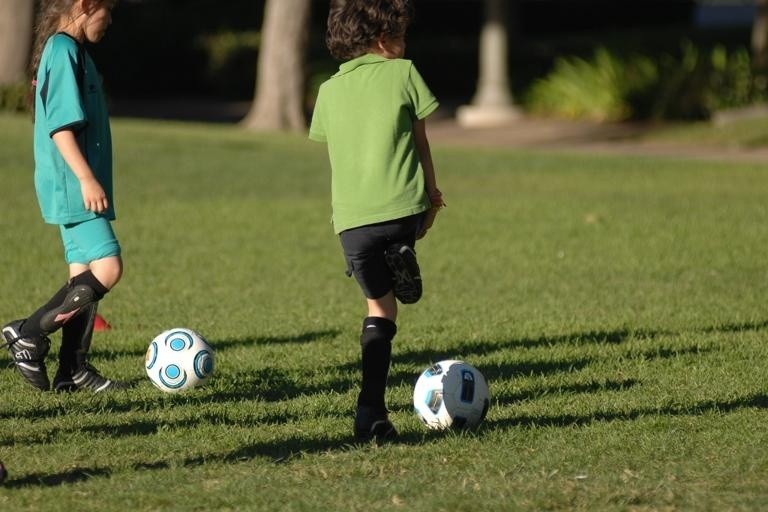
xmin=1 ymin=1 xmax=124 ymax=393
xmin=309 ymin=0 xmax=448 ymax=443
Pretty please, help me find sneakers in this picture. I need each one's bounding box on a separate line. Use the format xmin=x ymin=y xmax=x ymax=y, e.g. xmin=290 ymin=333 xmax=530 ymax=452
xmin=52 ymin=362 xmax=127 ymax=393
xmin=352 ymin=407 xmax=407 ymax=443
xmin=381 ymin=240 xmax=423 ymax=305
xmin=1 ymin=318 xmax=54 ymax=392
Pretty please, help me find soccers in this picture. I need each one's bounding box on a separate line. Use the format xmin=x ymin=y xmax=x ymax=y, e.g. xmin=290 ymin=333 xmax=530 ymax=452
xmin=145 ymin=328 xmax=215 ymax=394
xmin=413 ymin=360 xmax=490 ymax=432
